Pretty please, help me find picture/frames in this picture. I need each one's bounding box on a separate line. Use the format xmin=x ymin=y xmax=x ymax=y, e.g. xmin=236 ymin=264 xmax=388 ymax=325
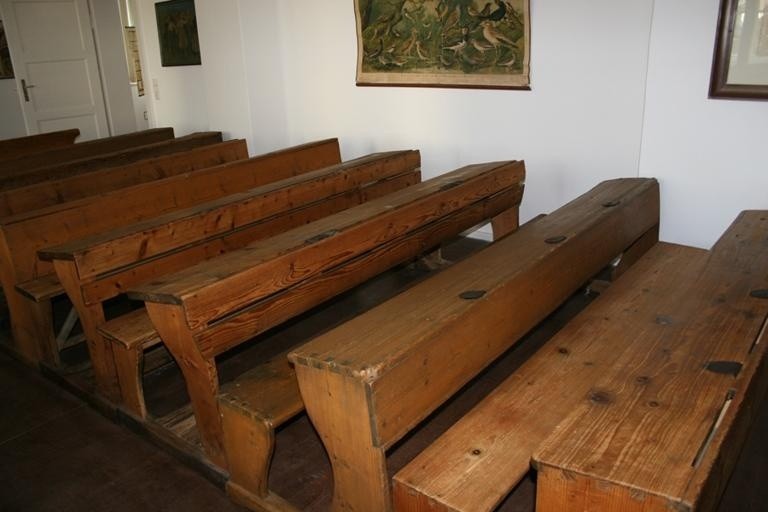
xmin=353 ymin=0 xmax=531 ymax=91
xmin=708 ymin=0 xmax=767 ymax=102
xmin=154 ymin=0 xmax=202 ymax=70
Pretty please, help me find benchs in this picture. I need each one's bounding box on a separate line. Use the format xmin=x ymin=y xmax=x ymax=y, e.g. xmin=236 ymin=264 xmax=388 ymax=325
xmin=0 ymin=128 xmax=768 ymax=512
